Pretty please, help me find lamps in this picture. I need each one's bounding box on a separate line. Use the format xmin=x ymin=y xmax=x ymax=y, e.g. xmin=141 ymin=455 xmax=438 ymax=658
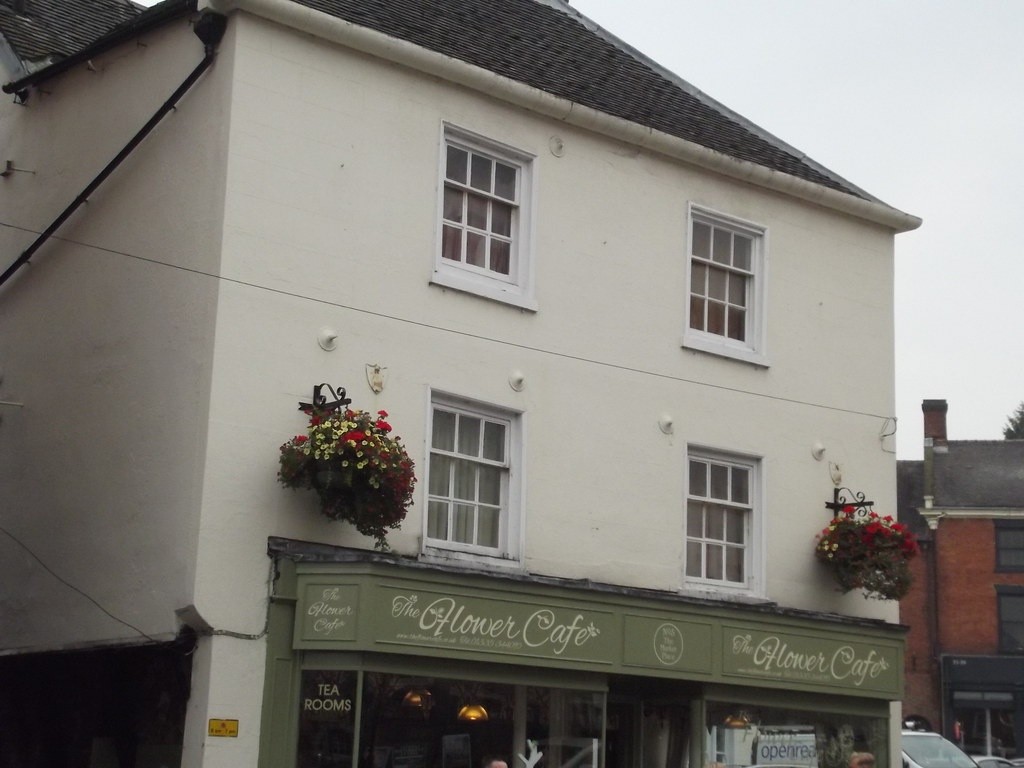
xmin=457 ymin=703 xmax=490 ymax=721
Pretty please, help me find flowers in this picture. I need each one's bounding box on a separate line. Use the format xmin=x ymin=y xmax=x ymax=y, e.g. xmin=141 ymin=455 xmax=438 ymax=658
xmin=275 ymin=406 xmax=418 ymax=550
xmin=812 ymin=502 xmax=918 ymax=601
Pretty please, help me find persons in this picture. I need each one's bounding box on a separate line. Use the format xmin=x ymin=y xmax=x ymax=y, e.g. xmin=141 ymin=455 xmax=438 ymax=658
xmin=850 ymin=752 xmax=876 ymax=768
xmin=481 ymin=756 xmax=508 ymax=768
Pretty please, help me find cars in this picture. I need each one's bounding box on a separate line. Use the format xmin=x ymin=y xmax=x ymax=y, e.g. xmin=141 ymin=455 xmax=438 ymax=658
xmin=901 ymin=729 xmax=1024 ymax=768
